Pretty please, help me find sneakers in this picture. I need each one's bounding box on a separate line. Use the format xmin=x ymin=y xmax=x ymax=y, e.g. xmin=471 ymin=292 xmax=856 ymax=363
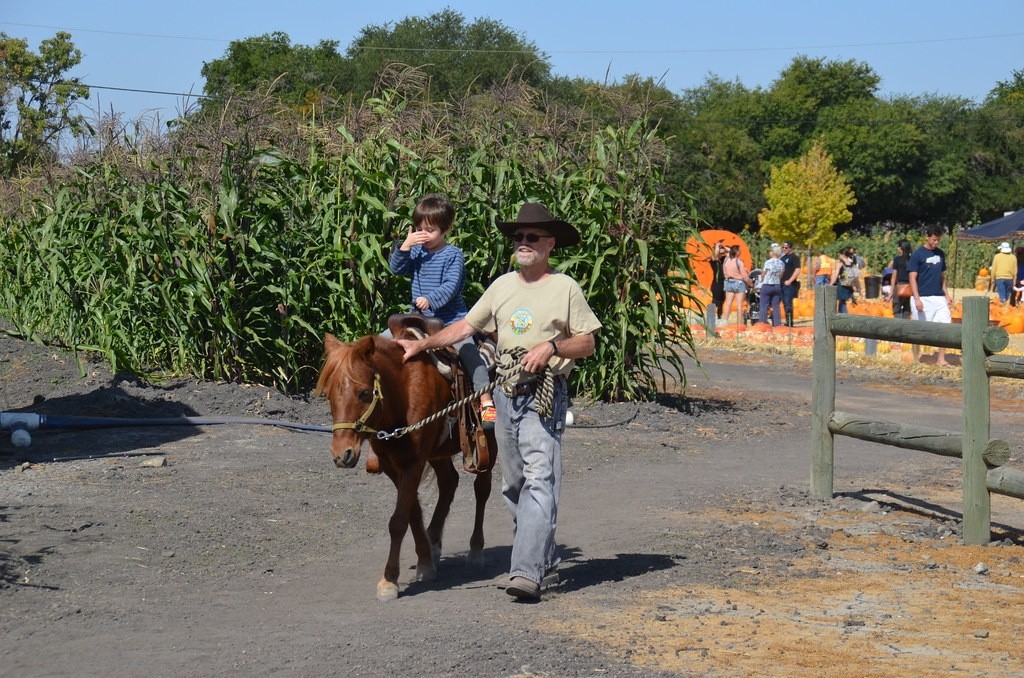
xmin=479 ymin=403 xmax=497 ymax=430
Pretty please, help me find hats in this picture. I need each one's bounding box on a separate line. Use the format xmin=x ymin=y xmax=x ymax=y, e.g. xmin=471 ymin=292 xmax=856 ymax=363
xmin=818 ymin=248 xmax=824 ymax=252
xmin=1015 ymin=247 xmax=1024 ymax=254
xmin=845 ymin=243 xmax=856 ymax=250
xmin=997 ymin=242 xmax=1012 ymax=253
xmin=495 ymin=202 xmax=579 ymax=248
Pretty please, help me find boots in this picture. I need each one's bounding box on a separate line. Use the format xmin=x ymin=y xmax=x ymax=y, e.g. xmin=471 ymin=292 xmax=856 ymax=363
xmin=785 ymin=312 xmax=792 ymax=326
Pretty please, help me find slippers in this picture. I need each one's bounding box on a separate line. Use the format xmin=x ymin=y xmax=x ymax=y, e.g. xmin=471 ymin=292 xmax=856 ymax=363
xmin=937 ymin=363 xmax=955 ymax=367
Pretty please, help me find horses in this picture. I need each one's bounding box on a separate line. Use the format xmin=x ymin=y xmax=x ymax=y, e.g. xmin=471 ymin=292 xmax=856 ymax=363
xmin=320 ymin=330 xmax=499 ymax=602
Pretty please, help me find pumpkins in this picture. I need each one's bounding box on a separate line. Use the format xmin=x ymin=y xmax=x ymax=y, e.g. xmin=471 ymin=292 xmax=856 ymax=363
xmin=647 ymin=269 xmax=1024 ymax=337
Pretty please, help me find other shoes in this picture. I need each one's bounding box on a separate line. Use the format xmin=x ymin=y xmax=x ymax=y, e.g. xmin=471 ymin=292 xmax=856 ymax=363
xmin=497 ymin=567 xmax=559 ymax=588
xmin=506 ymin=576 xmax=539 ymax=598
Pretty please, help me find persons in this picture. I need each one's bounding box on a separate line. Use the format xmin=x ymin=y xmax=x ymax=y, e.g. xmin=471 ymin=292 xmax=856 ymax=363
xmin=977 ymin=241 xmax=1024 ymax=309
xmin=880 ymin=226 xmax=961 ymax=370
xmin=388 ymin=205 xmax=600 ymax=602
xmin=375 ymin=195 xmax=500 ymax=428
xmin=707 ymin=238 xmax=868 ymax=329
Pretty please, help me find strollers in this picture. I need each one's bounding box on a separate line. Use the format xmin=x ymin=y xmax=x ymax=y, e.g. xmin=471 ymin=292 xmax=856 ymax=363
xmin=744 ymin=268 xmax=773 ymax=326
xmin=881 ymin=267 xmax=894 ymax=304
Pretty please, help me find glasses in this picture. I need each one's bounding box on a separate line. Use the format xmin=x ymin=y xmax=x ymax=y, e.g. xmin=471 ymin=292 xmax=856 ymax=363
xmin=782 ymin=247 xmax=788 ymax=249
xmin=512 ymin=233 xmax=552 ymax=242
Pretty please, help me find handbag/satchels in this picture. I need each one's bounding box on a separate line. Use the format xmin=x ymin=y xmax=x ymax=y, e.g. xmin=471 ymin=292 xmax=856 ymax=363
xmin=814 ymin=258 xmax=821 ymax=272
xmin=898 ymin=284 xmax=912 ymax=297
xmin=838 ymin=262 xmax=861 ymax=287
xmin=793 ymin=280 xmax=801 ymax=299
xmin=853 ymin=256 xmax=866 ymax=269
xmin=802 ymin=266 xmax=808 ymax=274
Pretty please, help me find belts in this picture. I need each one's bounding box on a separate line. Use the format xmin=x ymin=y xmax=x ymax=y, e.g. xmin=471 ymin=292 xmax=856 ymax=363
xmin=495 ymin=374 xmax=564 ymax=398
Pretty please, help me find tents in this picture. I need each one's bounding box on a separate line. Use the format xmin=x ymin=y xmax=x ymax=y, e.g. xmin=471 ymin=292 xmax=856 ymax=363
xmin=951 ymin=206 xmax=1024 ymax=302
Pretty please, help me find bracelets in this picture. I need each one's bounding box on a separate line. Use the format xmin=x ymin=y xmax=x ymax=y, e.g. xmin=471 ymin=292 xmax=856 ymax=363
xmin=547 ymin=340 xmax=558 ymax=355
